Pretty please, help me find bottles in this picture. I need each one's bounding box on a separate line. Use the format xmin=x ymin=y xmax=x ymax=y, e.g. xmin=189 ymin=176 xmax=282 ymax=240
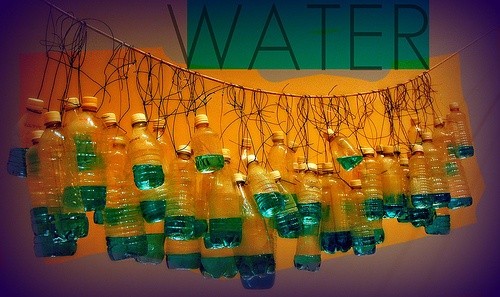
xmin=165 ymin=239 xmax=202 ymax=268
xmin=97 ymin=113 xmax=148 ymax=259
xmin=265 ymin=130 xmax=298 ymax=203
xmin=166 ymin=142 xmax=194 ymax=241
xmin=267 ymin=169 xmax=304 ymax=239
xmin=191 ymin=114 xmax=225 ymax=174
xmin=39 ymin=111 xmax=92 ymax=239
xmin=7 ymin=97 xmax=46 ymax=178
xmin=138 ymin=190 xmax=165 ymax=223
xmin=204 ymin=172 xmax=214 ymax=249
xmin=96 ymin=135 xmax=127 ymax=226
xmin=208 ymin=148 xmax=244 ymax=248
xmin=58 ymin=95 xmax=80 ymax=130
xmin=229 ymin=173 xmax=277 ymax=290
xmin=294 ymin=101 xmax=475 ymax=271
xmin=71 ymin=96 xmax=107 ymax=209
xmin=128 ymin=114 xmax=164 ymax=190
xmin=25 ymin=128 xmax=78 ymax=258
xmin=245 ymin=155 xmax=281 ymax=215
xmin=132 ymin=118 xmax=168 ymax=264
